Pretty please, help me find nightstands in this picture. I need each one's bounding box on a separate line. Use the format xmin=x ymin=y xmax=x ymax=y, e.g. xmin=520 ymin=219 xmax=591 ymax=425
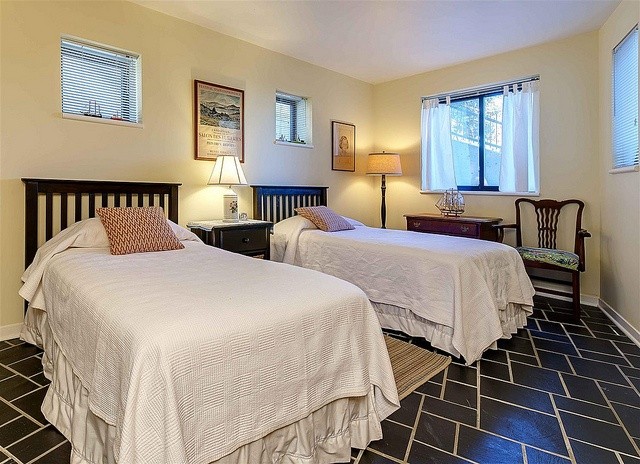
xmin=186 ymin=219 xmax=273 ymax=261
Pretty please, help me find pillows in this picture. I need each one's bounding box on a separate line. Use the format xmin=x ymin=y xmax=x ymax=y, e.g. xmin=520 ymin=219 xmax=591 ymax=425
xmin=96 ymin=205 xmax=186 ymax=255
xmin=293 ymin=206 xmax=356 ymax=233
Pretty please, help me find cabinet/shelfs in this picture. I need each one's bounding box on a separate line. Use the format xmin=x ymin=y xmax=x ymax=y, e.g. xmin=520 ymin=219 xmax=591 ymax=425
xmin=404 ymin=213 xmax=503 ymax=241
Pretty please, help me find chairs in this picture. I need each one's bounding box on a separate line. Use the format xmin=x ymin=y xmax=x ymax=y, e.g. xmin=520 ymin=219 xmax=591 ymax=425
xmin=492 ymin=197 xmax=591 ymax=315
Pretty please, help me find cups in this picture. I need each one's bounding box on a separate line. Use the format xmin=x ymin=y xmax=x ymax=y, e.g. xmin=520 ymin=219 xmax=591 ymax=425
xmin=239 ymin=212 xmax=247 ymax=221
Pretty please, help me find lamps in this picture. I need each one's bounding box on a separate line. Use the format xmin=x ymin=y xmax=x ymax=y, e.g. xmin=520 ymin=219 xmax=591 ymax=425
xmin=365 ymin=151 xmax=403 ymax=229
xmin=207 ymin=154 xmax=248 ymax=223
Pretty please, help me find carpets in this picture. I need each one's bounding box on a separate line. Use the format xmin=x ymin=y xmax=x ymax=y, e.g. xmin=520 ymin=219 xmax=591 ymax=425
xmin=383 ymin=332 xmax=453 ymax=403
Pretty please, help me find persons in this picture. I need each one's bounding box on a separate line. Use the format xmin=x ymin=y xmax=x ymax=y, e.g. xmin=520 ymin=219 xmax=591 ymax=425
xmin=339 ymin=135 xmax=348 ymax=156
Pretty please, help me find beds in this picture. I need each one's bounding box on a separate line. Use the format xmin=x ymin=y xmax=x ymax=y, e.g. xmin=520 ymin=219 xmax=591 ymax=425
xmin=250 ymin=184 xmax=535 ymax=365
xmin=20 ymin=176 xmax=402 ymax=464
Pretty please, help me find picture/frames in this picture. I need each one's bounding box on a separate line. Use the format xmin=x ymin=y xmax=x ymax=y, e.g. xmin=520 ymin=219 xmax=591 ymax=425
xmin=193 ymin=78 xmax=245 ymax=164
xmin=331 ymin=120 xmax=356 ymax=172
